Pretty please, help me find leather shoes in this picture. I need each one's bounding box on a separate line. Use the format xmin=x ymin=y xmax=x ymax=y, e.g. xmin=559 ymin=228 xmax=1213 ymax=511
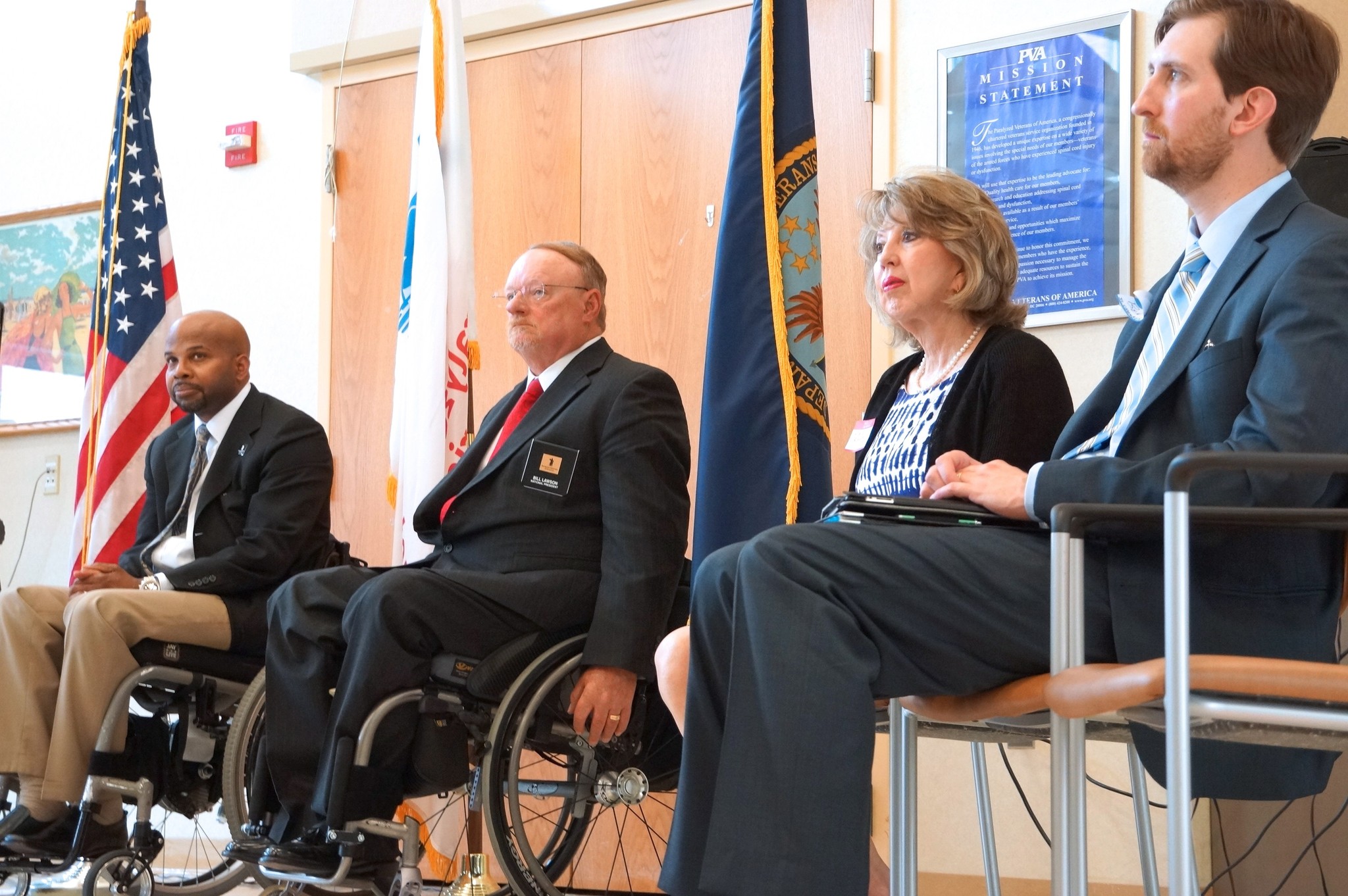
xmin=4 ymin=809 xmax=129 ymax=858
xmin=222 ymin=826 xmax=298 ymax=867
xmin=0 ymin=803 xmax=49 ymax=861
xmin=257 ymin=828 xmax=378 ymax=878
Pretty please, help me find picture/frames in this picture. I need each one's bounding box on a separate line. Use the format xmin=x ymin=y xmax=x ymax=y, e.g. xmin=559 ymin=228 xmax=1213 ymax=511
xmin=0 ymin=200 xmax=102 ymax=438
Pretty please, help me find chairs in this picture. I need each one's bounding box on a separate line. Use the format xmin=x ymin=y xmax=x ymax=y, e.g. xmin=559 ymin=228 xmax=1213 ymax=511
xmin=865 ymin=452 xmax=1348 ymax=896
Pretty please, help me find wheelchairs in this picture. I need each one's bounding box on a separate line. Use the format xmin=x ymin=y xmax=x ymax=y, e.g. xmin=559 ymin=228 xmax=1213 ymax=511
xmin=221 ymin=557 xmax=690 ymax=896
xmin=0 ymin=533 xmax=368 ymax=896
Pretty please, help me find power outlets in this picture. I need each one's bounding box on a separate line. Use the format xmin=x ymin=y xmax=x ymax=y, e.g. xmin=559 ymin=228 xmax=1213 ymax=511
xmin=44 ymin=454 xmax=60 ymax=495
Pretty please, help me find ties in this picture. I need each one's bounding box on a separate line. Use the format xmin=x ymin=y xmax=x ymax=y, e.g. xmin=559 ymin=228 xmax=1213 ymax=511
xmin=440 ymin=377 xmax=544 ymax=525
xmin=1056 ymin=243 xmax=1208 ymax=459
xmin=139 ymin=423 xmax=212 ymax=575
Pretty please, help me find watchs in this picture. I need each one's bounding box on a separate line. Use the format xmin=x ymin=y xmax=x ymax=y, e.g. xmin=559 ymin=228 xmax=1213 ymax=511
xmin=139 ymin=575 xmax=160 ymax=591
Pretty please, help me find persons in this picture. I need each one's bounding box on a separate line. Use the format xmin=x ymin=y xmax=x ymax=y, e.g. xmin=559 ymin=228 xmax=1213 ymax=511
xmin=659 ymin=0 xmax=1348 ymax=896
xmin=0 ymin=310 xmax=334 ymax=861
xmin=655 ymin=169 xmax=1077 ymax=896
xmin=221 ymin=239 xmax=691 ymax=880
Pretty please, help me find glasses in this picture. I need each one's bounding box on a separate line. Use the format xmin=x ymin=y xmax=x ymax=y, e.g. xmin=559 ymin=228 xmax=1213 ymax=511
xmin=490 ymin=279 xmax=590 ymax=307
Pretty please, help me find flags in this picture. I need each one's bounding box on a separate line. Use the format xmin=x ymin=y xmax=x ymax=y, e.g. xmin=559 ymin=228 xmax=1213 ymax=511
xmin=66 ymin=11 xmax=184 ymax=592
xmin=386 ymin=0 xmax=480 ymax=882
xmin=689 ymin=0 xmax=835 ymax=578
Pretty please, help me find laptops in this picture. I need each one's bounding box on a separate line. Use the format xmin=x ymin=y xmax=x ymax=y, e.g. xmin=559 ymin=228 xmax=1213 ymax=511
xmin=822 ymin=492 xmax=1043 ymax=534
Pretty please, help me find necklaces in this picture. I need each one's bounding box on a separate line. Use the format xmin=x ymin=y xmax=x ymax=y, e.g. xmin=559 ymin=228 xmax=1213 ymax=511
xmin=914 ymin=321 xmax=983 ymax=392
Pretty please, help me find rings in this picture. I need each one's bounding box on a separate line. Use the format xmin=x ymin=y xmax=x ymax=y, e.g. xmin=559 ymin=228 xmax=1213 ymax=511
xmin=957 ymin=472 xmax=963 ymax=478
xmin=609 ymin=714 xmax=621 ymax=721
